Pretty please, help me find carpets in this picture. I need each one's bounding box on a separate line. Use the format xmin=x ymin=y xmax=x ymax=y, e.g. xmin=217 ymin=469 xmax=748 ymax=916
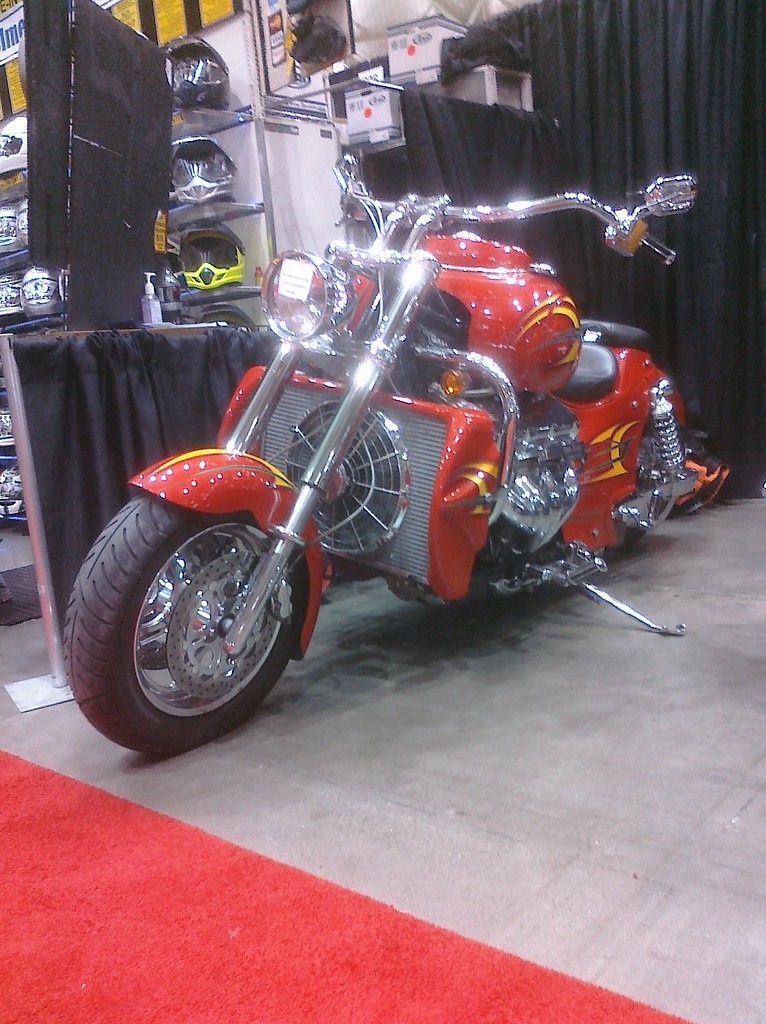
xmin=0 ymin=749 xmax=685 ymax=1024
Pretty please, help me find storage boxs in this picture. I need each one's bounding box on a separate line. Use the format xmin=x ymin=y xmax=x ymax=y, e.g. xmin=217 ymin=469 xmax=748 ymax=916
xmin=344 ymin=79 xmax=402 ymax=147
xmin=386 ymin=12 xmax=467 ymax=86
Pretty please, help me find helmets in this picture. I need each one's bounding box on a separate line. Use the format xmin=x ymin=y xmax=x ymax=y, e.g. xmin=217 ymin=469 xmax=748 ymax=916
xmin=164 ymin=36 xmax=229 ymax=111
xmin=178 ymin=219 xmax=248 ymax=290
xmin=0 ymin=117 xmax=69 ymax=520
xmin=170 ymin=135 xmax=235 ymax=203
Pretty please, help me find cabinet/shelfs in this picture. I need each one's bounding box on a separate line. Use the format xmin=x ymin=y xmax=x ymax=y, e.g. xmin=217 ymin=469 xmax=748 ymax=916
xmin=156 ymin=105 xmax=276 ymax=325
xmin=0 ymin=0 xmax=170 ymax=519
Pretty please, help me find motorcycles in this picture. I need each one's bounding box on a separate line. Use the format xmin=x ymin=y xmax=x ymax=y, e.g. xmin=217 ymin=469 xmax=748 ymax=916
xmin=63 ymin=154 xmax=698 ymax=759
